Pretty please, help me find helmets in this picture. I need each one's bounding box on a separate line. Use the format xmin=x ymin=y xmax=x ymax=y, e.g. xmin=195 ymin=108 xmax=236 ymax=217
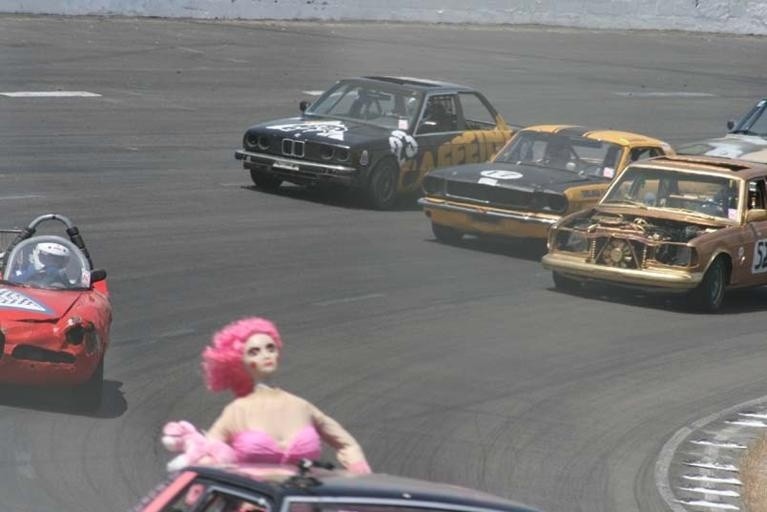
xmin=406 ymin=97 xmax=433 ymax=122
xmin=32 ymin=242 xmax=70 ymax=273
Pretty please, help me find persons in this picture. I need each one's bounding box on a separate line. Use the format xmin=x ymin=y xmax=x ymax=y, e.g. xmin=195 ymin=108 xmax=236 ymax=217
xmin=160 ymin=317 xmax=373 ymax=512
xmin=10 ymin=242 xmax=71 ymax=286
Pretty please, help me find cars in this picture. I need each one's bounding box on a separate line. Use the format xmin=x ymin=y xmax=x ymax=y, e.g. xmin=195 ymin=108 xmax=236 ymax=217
xmin=631 ymin=99 xmax=767 ymax=167
xmin=541 ymin=153 xmax=765 ymax=313
xmin=0 ymin=212 xmax=109 ymax=412
xmin=133 ymin=463 xmax=539 ymax=512
xmin=234 ymin=74 xmax=525 ymax=204
xmin=420 ymin=125 xmax=674 ymax=260
